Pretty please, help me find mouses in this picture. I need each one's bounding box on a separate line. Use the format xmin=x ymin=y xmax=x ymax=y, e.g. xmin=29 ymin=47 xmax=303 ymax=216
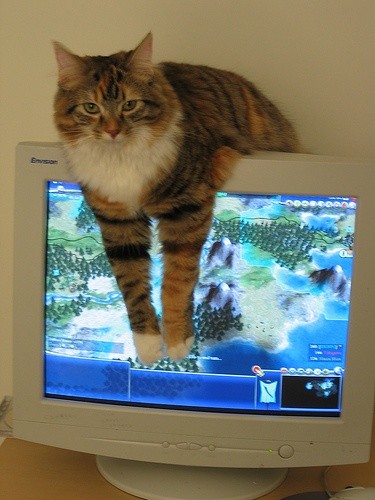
xmin=328 ymin=484 xmax=375 ymax=500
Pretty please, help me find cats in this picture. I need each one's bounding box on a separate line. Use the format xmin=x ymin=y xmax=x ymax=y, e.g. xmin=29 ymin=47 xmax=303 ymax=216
xmin=49 ymin=29 xmax=301 ymax=366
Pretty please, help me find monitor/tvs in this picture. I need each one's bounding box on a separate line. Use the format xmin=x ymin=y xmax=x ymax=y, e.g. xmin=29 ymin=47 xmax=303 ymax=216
xmin=7 ymin=140 xmax=375 ymax=466
